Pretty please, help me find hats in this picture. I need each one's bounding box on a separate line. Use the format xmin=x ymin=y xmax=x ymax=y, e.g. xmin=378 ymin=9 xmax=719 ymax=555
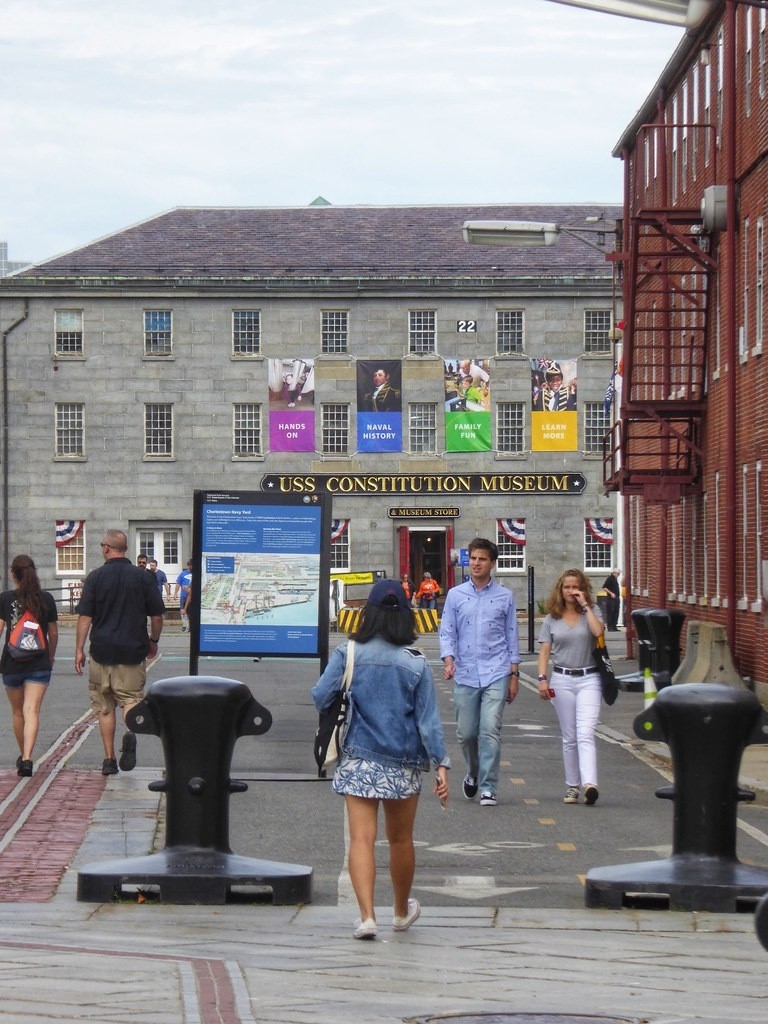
xmin=368 ymin=579 xmax=407 ymax=610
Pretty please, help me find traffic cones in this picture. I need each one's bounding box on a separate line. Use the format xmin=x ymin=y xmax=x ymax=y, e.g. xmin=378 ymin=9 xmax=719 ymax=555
xmin=642 ymin=667 xmax=660 ymax=729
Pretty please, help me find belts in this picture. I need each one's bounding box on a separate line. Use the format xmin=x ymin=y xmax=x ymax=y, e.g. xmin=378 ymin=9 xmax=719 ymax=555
xmin=554 ymin=666 xmax=599 ymax=677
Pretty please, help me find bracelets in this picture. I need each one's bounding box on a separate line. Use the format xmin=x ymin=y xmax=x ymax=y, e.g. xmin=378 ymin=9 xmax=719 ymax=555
xmin=150 ymin=635 xmax=161 ymax=644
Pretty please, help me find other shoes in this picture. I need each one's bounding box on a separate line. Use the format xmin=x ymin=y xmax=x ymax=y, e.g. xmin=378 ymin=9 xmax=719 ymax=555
xmin=608 ymin=627 xmax=620 ymax=632
xmin=182 ymin=627 xmax=186 ymax=631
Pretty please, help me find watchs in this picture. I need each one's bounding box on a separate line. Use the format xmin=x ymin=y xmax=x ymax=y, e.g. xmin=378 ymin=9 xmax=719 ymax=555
xmin=509 ymin=669 xmax=520 ymax=676
xmin=538 ymin=674 xmax=545 ymax=680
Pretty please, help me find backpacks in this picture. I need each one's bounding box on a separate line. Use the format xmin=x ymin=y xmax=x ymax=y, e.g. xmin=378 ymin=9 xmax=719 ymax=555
xmin=8 ymin=592 xmax=46 ymax=661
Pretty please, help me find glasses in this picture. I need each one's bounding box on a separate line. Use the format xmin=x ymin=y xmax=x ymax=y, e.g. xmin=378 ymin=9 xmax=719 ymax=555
xmin=101 ymin=543 xmax=110 ymax=547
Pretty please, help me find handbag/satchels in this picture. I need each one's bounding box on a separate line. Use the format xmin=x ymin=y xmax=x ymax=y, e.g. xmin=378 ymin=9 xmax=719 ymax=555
xmin=591 ymin=636 xmax=618 ymax=706
xmin=314 ymin=640 xmax=354 ymax=766
xmin=432 ymin=592 xmax=439 ymax=598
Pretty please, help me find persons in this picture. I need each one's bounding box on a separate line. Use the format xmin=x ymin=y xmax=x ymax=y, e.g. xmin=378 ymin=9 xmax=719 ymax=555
xmin=533 ymin=361 xmax=577 ymax=411
xmin=76 ymin=529 xmax=166 ymax=784
xmin=282 ymin=372 xmax=313 ymax=407
xmin=137 ymin=553 xmax=171 ymax=603
xmin=400 ymin=572 xmax=439 ymax=609
xmin=311 ymin=580 xmax=452 ymax=939
xmin=454 ymin=360 xmax=490 ymax=405
xmin=602 ymin=567 xmax=622 ymax=632
xmin=537 ymin=570 xmax=605 ymax=805
xmin=0 ymin=554 xmax=58 ymax=777
xmin=439 ymin=536 xmax=521 ymax=806
xmin=364 ymin=368 xmax=401 ymax=412
xmin=173 ymin=559 xmax=192 ymax=632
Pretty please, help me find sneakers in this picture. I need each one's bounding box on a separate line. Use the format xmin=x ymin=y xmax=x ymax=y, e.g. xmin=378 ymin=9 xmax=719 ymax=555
xmin=462 ymin=775 xmax=478 ymax=800
xmin=583 ymin=784 xmax=599 ymax=804
xmin=16 ymin=754 xmax=32 ymax=776
xmin=392 ymin=898 xmax=420 ymax=931
xmin=353 ymin=917 xmax=377 ymax=938
xmin=101 ymin=758 xmax=118 ymax=775
xmin=119 ymin=731 xmax=136 ymax=770
xmin=480 ymin=791 xmax=497 ymax=806
xmin=564 ymin=786 xmax=581 ymax=803
